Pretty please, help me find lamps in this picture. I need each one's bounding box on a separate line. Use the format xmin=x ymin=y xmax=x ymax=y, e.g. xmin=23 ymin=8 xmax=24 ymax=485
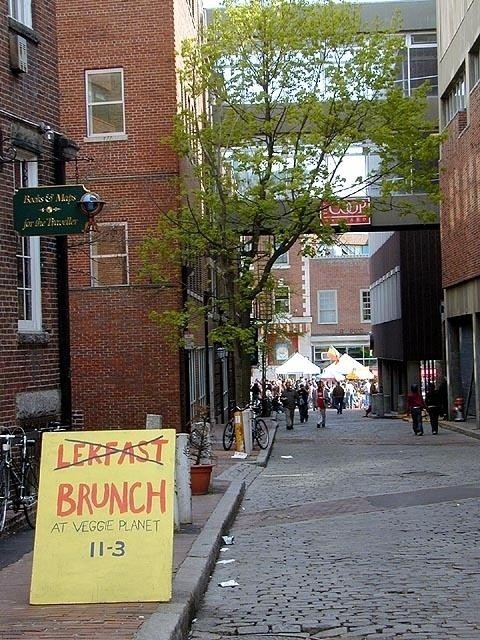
xmin=54 ymin=138 xmax=80 ymax=162
xmin=76 ymin=191 xmax=107 ymax=233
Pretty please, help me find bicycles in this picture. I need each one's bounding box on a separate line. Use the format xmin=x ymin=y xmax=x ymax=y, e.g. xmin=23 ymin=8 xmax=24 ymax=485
xmin=0 ymin=434 xmax=39 ymax=535
xmin=222 ymin=403 xmax=258 ymax=451
xmin=230 ymin=404 xmax=270 ymax=450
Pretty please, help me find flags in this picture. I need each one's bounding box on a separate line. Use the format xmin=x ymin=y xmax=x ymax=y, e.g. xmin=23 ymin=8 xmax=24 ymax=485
xmin=327 ymin=345 xmax=342 ymax=361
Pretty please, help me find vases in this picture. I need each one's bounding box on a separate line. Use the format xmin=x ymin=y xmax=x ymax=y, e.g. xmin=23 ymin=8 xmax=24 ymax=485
xmin=191 ymin=464 xmax=216 ymax=496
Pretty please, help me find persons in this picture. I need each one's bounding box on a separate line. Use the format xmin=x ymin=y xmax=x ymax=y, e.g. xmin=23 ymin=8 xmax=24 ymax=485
xmin=406 ymin=384 xmax=426 ymax=436
xmin=250 ymin=376 xmax=379 ymax=430
xmin=424 ymin=383 xmax=443 ymax=435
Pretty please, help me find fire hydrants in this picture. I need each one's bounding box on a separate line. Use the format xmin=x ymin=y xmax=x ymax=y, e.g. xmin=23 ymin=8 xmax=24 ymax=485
xmin=453 ymin=397 xmax=465 ymax=422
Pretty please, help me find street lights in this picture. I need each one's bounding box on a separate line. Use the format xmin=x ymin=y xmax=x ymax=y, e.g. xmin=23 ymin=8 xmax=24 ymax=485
xmin=49 ymin=131 xmax=80 ymax=431
